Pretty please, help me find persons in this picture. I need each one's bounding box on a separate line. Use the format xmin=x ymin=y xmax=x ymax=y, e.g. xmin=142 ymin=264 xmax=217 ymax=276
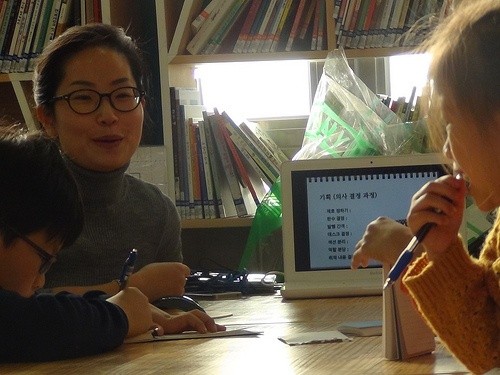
xmin=0 ymin=128 xmax=227 ymax=364
xmin=351 ymin=1 xmax=500 ymax=374
xmin=32 ymin=23 xmax=192 ymax=303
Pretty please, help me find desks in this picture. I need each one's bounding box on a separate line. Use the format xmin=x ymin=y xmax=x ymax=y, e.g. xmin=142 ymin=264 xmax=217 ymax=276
xmin=0 ymin=290 xmax=500 ymax=375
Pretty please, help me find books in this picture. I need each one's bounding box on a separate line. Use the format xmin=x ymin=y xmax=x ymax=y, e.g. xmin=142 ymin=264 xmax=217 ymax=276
xmin=380 ymin=217 xmax=436 ymax=361
xmin=0 ymin=0 xmax=102 ymax=73
xmin=185 ymin=0 xmax=466 ymax=56
xmin=329 ymin=87 xmax=457 ymax=159
xmin=336 ymin=320 xmax=383 ymax=337
xmin=170 ymin=87 xmax=295 ymax=219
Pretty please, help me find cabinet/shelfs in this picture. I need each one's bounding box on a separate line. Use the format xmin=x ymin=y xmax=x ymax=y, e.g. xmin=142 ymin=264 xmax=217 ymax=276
xmin=155 ymin=0 xmax=432 ymax=228
xmin=0 ymin=0 xmax=144 ymax=132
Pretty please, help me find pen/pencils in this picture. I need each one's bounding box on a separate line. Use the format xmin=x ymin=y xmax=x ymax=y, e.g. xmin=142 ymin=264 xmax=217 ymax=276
xmin=382 ymin=197 xmax=443 ymax=293
xmin=117 ymin=248 xmax=137 ymax=293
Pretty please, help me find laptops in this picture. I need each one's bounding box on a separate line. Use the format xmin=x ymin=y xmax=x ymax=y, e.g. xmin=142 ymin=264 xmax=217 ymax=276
xmin=280 ymin=153 xmax=466 ymax=298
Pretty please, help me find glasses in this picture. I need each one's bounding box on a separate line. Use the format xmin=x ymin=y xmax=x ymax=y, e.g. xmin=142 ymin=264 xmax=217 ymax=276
xmin=18 ymin=234 xmax=57 ymax=274
xmin=46 ymin=85 xmax=147 ymax=115
